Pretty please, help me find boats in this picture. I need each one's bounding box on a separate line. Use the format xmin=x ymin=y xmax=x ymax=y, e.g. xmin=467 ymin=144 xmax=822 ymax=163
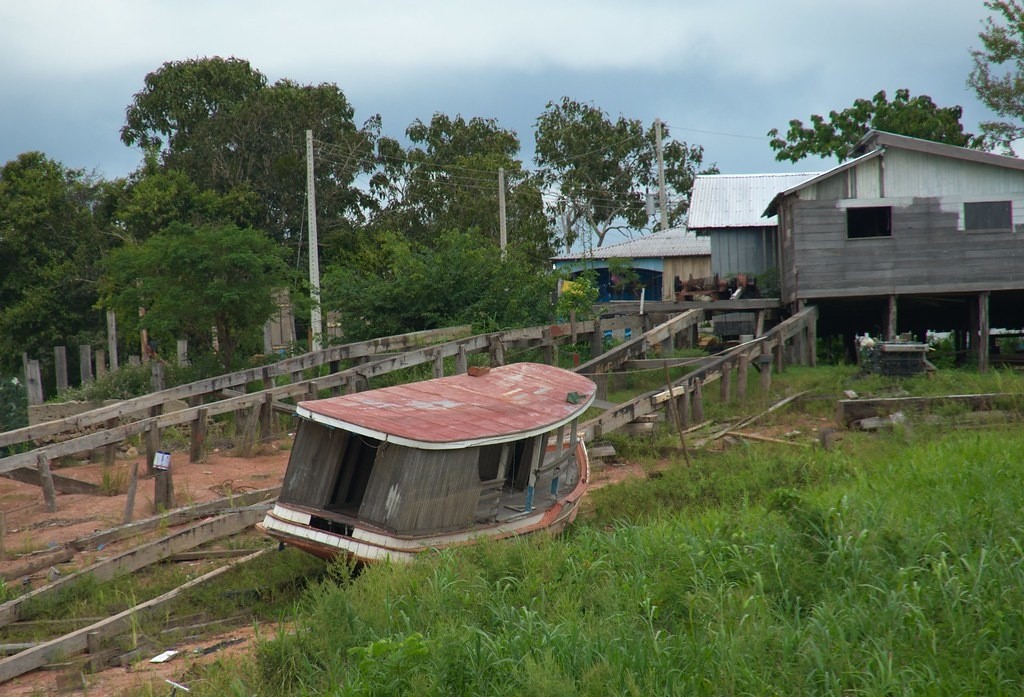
xmin=252 ymin=348 xmax=600 ymax=576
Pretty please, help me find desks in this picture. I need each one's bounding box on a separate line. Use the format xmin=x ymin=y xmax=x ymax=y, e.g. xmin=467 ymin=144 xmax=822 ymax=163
xmin=873 ymin=344 xmax=929 ymax=376
